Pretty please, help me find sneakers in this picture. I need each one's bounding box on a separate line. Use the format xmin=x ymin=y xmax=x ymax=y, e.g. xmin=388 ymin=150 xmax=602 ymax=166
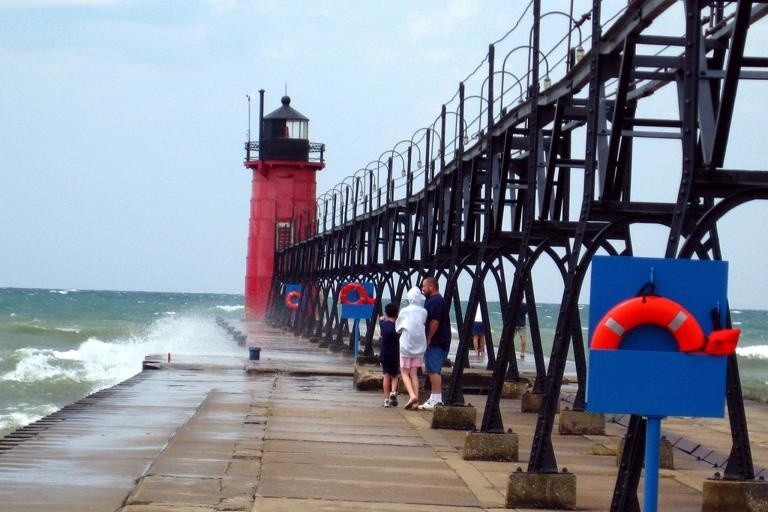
xmin=418 ymin=401 xmax=444 ymax=411
xmin=384 ymin=392 xmax=398 ymax=408
xmin=404 ymin=398 xmax=418 ymax=410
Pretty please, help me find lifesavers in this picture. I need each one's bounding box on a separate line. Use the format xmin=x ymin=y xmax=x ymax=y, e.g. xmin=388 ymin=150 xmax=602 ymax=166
xmin=339 ymin=282 xmax=368 ymax=304
xmin=590 ymin=294 xmax=706 ymax=356
xmin=285 ymin=291 xmax=301 ymax=310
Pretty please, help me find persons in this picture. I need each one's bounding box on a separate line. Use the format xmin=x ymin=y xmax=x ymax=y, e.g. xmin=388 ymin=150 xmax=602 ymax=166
xmin=395 ymin=287 xmax=428 ymax=409
xmin=472 ymin=301 xmax=485 ymax=357
xmin=379 ymin=303 xmax=401 ymax=407
xmin=418 ymin=277 xmax=451 ymax=411
xmin=513 ymin=293 xmax=528 ymax=357
